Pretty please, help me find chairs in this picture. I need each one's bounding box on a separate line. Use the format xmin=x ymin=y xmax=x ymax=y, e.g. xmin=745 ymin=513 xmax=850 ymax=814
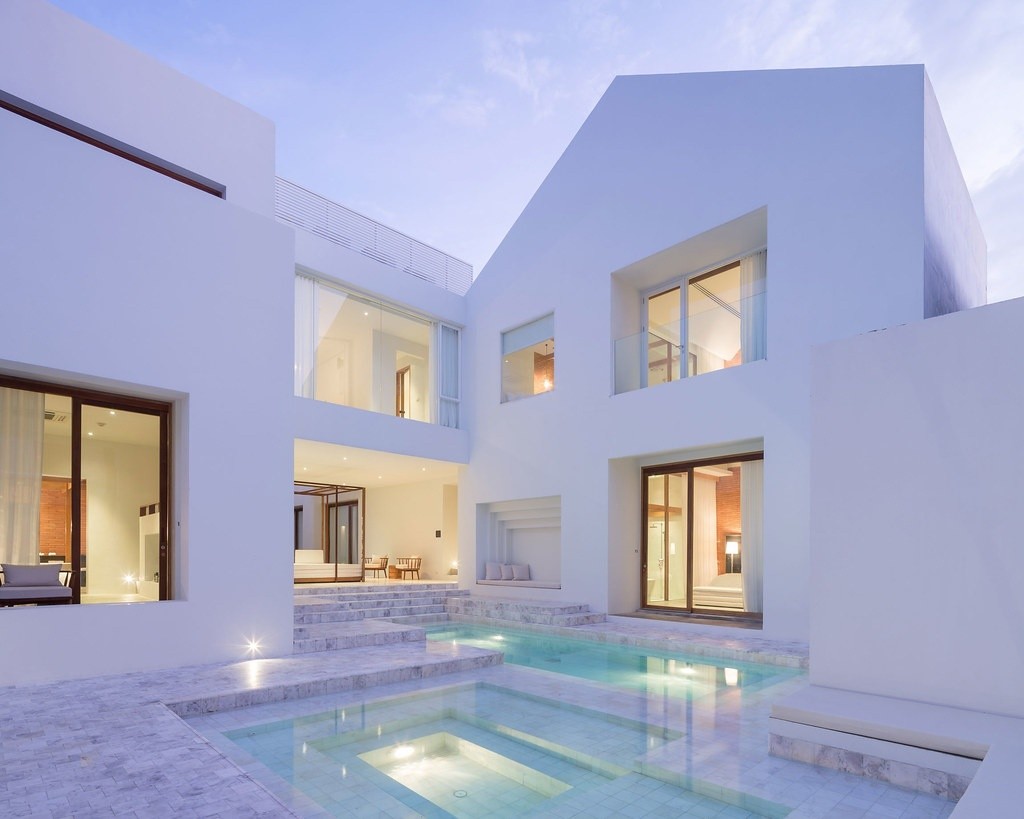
xmin=0 ymin=563 xmax=76 ymax=608
xmin=396 ymin=558 xmax=422 ymax=580
xmin=361 ymin=557 xmax=389 ymax=578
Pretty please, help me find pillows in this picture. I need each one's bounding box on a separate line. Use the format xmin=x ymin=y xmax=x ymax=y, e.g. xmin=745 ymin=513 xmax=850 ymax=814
xmin=502 ymin=391 xmax=521 ymax=402
xmin=512 ymin=565 xmax=530 ymax=581
xmin=500 ymin=564 xmax=514 ymax=580
xmin=486 ymin=561 xmax=502 ymax=579
xmin=372 ymin=552 xmax=388 ymax=564
xmin=407 ymin=553 xmax=422 ymax=566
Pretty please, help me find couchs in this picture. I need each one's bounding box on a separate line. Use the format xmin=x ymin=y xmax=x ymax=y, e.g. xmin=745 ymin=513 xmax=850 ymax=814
xmin=693 ymin=573 xmax=743 ymax=608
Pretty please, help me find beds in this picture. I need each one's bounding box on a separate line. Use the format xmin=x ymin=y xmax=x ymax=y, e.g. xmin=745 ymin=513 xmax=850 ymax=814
xmin=294 ymin=550 xmax=364 ymax=583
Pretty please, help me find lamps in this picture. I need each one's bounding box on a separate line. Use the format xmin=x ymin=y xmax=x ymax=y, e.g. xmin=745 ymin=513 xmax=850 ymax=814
xmin=725 ymin=541 xmax=738 ymax=573
